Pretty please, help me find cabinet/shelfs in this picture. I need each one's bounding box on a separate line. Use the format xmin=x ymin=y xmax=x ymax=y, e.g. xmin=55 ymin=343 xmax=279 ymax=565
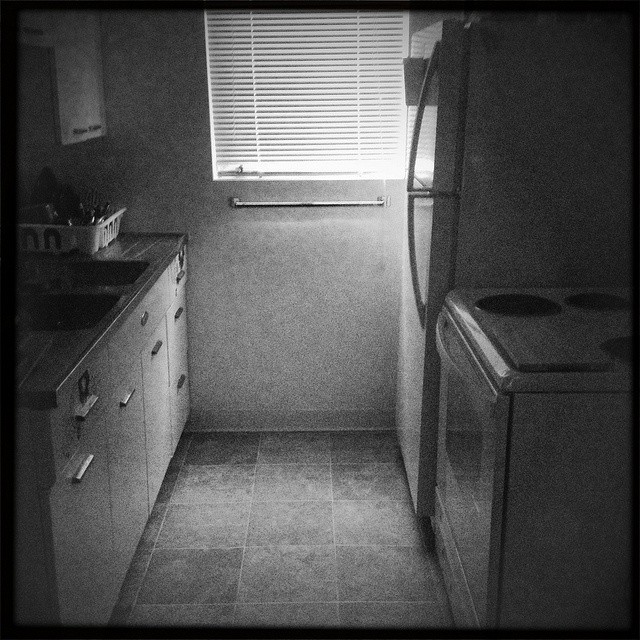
xmin=19 ymin=5 xmax=106 ymax=145
xmin=108 ymin=302 xmax=150 ymax=588
xmin=140 ymin=268 xmax=172 ymax=517
xmin=13 ymin=341 xmax=113 ymax=626
xmin=166 ymin=246 xmax=191 ymax=458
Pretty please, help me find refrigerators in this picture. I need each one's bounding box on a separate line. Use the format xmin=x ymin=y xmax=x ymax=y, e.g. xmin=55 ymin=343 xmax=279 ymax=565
xmin=396 ymin=20 xmax=631 ymax=537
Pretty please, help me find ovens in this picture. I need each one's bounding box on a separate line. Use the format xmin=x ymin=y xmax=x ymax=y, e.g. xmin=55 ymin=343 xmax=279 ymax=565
xmin=430 ymin=306 xmax=507 ymax=617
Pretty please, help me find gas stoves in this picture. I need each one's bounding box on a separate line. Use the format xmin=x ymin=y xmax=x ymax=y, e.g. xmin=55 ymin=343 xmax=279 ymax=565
xmin=449 ymin=286 xmax=636 ymax=386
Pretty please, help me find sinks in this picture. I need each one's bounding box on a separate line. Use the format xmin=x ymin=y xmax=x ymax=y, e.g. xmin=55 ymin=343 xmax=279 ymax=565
xmin=24 ymin=259 xmax=151 ymax=288
xmin=17 ymin=290 xmax=121 ymax=332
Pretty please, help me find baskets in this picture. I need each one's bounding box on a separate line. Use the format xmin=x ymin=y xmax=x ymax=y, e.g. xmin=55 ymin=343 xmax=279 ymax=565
xmin=15 ymin=200 xmax=126 ymax=253
